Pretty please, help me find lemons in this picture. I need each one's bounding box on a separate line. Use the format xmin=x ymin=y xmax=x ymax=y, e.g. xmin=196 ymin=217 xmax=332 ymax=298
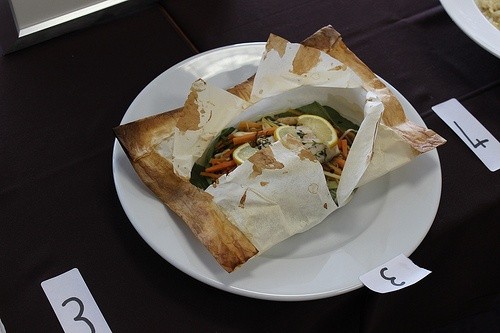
xmin=232 ymin=113 xmax=338 ymax=164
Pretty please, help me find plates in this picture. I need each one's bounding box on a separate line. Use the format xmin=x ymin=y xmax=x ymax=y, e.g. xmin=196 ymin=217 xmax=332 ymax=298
xmin=113 ymin=41 xmax=444 ymax=301
xmin=440 ymin=0 xmax=500 ymax=57
